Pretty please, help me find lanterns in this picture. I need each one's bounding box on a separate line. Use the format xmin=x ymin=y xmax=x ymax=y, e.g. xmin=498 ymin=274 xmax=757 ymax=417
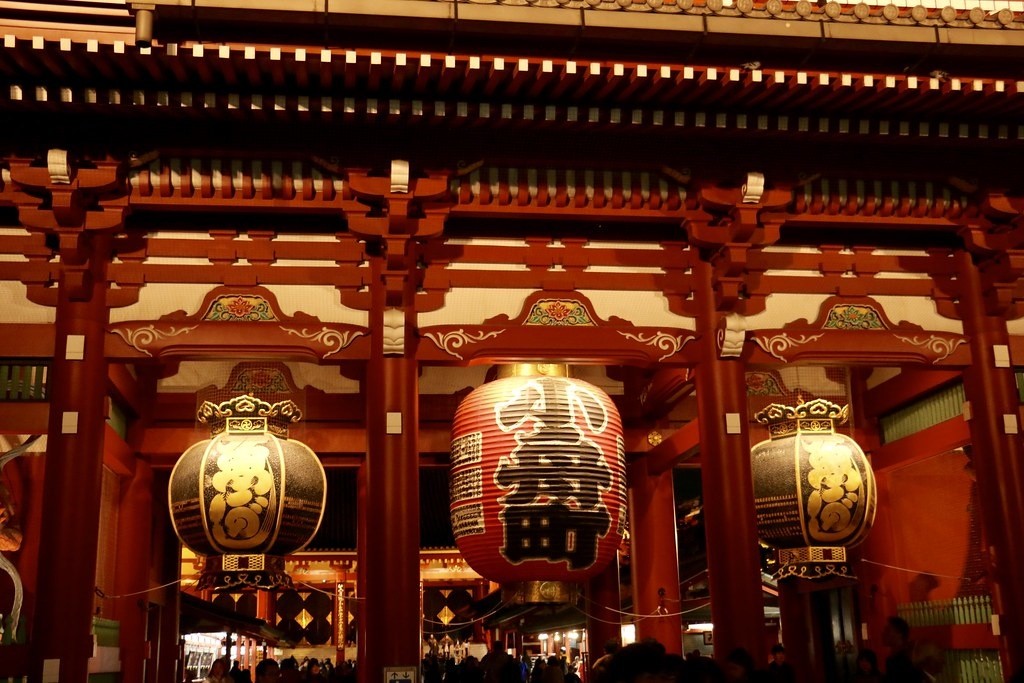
xmin=749 ymin=396 xmax=877 ymax=582
xmin=168 ymin=394 xmax=328 ymax=590
xmin=447 ymin=359 xmax=627 ymax=604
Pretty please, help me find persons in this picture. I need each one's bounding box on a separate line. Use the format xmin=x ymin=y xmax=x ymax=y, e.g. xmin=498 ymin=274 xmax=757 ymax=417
xmin=204 ymin=652 xmax=356 ymax=683
xmin=593 ymin=637 xmax=795 ymax=683
xmin=421 ymin=641 xmax=582 ymax=683
